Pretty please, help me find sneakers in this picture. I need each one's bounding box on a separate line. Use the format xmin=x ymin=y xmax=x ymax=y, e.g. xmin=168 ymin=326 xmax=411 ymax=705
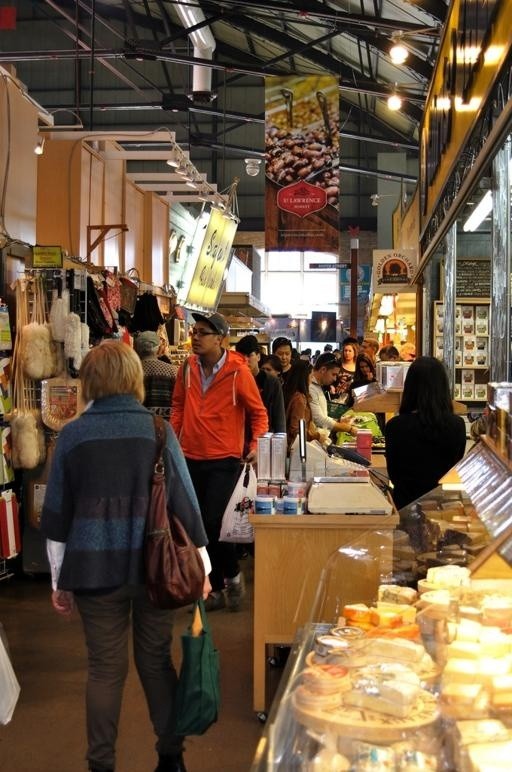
xmin=226 ymin=571 xmax=245 ymax=611
xmin=188 ymin=591 xmax=225 ymax=613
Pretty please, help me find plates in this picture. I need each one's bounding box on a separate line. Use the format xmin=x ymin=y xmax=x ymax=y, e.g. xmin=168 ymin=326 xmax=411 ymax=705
xmin=414 ymin=551 xmax=475 ymax=575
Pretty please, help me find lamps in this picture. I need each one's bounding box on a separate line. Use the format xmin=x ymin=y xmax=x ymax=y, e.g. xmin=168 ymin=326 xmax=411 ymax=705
xmin=34 ymin=138 xmax=45 ymax=155
xmin=87 ymin=131 xmax=228 ymax=205
xmin=370 ymin=194 xmax=400 ymax=206
xmin=245 ymin=158 xmax=262 ymax=176
xmin=389 ymin=26 xmax=438 ymax=66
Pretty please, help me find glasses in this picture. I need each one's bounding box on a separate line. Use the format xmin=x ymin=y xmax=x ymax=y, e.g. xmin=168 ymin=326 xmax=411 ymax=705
xmin=322 ymin=357 xmax=337 ymax=365
xmin=193 ymin=329 xmax=217 ymax=336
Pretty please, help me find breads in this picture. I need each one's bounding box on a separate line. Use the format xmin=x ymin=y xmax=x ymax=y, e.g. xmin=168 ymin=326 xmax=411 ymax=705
xmin=295 ymin=485 xmax=510 ymax=772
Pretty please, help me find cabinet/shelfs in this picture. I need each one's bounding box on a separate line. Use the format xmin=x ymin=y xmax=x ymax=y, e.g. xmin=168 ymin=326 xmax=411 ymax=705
xmin=263 ymin=443 xmax=512 ymax=771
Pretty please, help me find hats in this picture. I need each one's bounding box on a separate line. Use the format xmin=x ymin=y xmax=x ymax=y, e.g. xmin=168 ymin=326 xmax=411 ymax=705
xmin=136 ymin=331 xmax=160 ymax=346
xmin=192 ymin=313 xmax=228 ymax=336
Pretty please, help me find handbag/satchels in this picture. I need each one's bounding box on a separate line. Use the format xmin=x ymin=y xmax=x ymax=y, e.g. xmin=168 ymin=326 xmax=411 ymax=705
xmin=175 ymin=625 xmax=221 ymax=736
xmin=146 ymin=474 xmax=205 ymax=610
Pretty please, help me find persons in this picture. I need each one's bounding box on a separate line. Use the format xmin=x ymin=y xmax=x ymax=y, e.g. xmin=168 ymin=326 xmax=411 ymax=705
xmin=385 ymin=356 xmax=466 ymax=508
xmin=168 ymin=313 xmax=271 ymax=615
xmin=132 ymin=331 xmax=413 ymax=467
xmin=39 ymin=339 xmax=213 ymax=772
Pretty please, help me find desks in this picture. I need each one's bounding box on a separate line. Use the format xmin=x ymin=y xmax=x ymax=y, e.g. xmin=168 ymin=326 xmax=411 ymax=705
xmin=252 ymin=479 xmax=401 ymax=720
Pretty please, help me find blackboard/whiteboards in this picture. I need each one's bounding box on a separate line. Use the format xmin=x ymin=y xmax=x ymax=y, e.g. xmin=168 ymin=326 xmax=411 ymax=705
xmin=439 ymin=257 xmax=491 ymax=300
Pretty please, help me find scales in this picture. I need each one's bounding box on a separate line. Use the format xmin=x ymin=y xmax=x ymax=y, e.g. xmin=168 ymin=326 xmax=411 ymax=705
xmin=307 ymin=476 xmax=392 ymax=515
